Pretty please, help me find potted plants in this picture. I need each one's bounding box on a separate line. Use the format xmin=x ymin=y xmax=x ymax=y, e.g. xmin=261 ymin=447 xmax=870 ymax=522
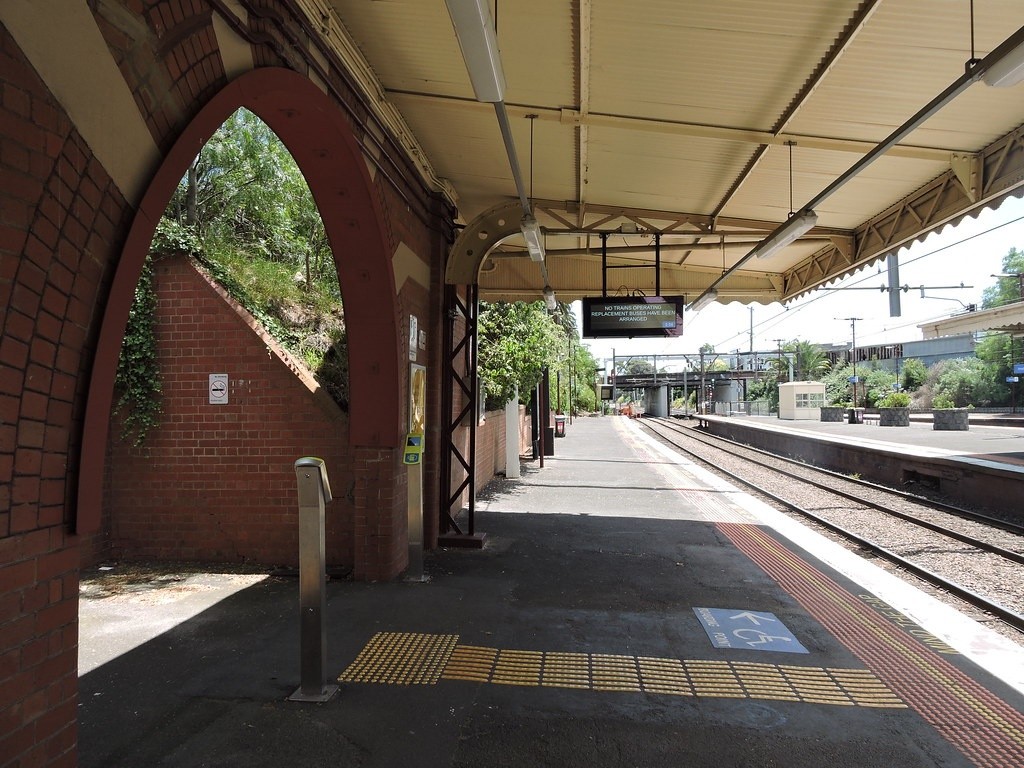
xmin=820 ymin=403 xmax=845 ymax=422
xmin=931 ymin=394 xmax=976 ymax=431
xmin=873 ymin=392 xmax=910 ymax=427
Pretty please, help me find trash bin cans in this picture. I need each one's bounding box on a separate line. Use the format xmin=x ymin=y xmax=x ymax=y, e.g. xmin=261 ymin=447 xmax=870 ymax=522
xmin=554 ymin=415 xmax=566 ymax=437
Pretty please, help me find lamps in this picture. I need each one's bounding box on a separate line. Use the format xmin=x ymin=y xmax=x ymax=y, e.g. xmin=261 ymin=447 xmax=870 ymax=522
xmin=984 ymin=41 xmax=1024 ymax=88
xmin=756 ymin=210 xmax=818 ymax=261
xmin=692 ymin=289 xmax=718 ymax=311
xmin=520 ymin=212 xmax=546 ymax=262
xmin=542 ymin=286 xmax=557 ymax=310
xmin=443 ymin=0 xmax=506 ymax=102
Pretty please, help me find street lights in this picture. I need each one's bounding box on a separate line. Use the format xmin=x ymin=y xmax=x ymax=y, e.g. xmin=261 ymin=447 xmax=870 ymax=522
xmin=835 ymin=317 xmax=875 ymax=407
